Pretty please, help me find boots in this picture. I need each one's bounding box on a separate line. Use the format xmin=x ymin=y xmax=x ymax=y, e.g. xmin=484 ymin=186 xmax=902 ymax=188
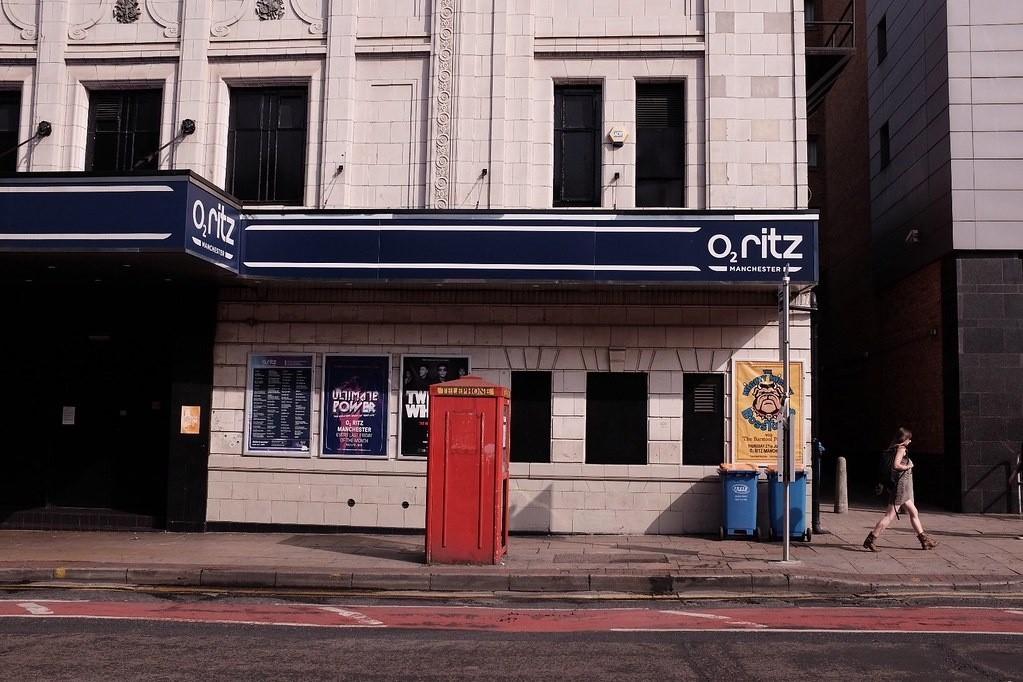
xmin=916 ymin=530 xmax=939 ymax=550
xmin=863 ymin=531 xmax=881 ymax=552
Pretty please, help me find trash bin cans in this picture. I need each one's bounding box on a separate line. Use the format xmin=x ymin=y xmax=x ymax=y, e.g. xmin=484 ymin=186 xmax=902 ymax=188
xmin=765 ymin=464 xmax=808 ymax=536
xmin=719 ymin=463 xmax=761 ymax=538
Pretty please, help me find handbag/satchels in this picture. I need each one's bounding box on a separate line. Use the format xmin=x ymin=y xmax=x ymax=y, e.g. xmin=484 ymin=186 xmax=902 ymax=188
xmin=872 ymin=446 xmax=909 ymax=506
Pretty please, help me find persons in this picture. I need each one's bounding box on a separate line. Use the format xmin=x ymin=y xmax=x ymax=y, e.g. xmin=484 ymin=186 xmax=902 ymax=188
xmin=862 ymin=427 xmax=940 ymax=552
xmin=404 ymin=365 xmax=467 ymax=452
